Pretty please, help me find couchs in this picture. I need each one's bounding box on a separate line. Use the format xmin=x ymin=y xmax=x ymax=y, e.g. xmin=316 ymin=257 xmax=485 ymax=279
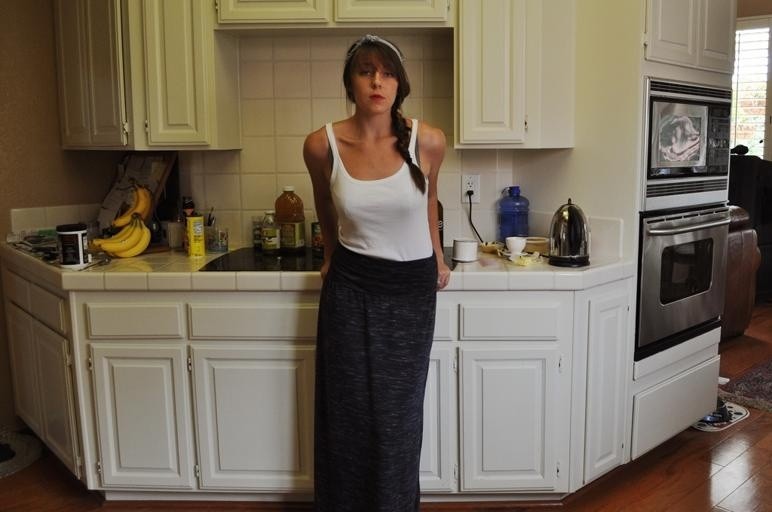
xmin=721 ymin=205 xmax=761 ymax=348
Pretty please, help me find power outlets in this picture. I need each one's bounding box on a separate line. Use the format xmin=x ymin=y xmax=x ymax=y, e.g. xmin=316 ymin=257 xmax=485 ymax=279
xmin=462 ymin=175 xmax=480 ymax=203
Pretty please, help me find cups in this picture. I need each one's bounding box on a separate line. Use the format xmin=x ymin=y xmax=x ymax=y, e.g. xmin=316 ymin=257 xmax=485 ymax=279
xmin=453 ymin=238 xmax=478 ymax=259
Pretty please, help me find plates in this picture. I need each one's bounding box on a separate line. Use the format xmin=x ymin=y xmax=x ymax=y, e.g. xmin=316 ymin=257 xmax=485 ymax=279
xmin=452 ymin=258 xmax=480 ymax=262
xmin=503 ymin=252 xmax=527 ymax=256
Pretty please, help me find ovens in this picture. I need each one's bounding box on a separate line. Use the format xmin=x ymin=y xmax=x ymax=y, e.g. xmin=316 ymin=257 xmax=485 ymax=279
xmin=635 ymin=70 xmax=733 ymax=361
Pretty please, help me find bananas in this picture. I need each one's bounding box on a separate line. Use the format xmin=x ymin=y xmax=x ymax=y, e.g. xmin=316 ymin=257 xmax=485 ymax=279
xmin=110 ymin=176 xmax=152 ymax=226
xmin=88 ymin=212 xmax=151 ymax=258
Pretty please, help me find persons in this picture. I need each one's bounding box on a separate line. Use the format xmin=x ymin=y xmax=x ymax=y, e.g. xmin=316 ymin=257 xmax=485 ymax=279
xmin=303 ymin=35 xmax=450 ymax=510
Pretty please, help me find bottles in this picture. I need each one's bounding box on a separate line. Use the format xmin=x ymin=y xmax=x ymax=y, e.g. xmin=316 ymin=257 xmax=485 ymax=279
xmin=54 ymin=222 xmax=93 ymax=270
xmin=252 ymin=185 xmax=324 ymax=254
xmin=499 ymin=186 xmax=531 ymax=243
xmin=152 ymin=194 xmax=230 ymax=253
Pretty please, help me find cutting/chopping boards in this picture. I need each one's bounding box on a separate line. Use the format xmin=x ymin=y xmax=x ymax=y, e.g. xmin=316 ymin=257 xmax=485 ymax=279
xmin=94 ymin=149 xmax=177 ymax=232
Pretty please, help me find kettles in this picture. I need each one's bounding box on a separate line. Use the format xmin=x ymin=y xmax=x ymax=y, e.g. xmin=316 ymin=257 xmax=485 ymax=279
xmin=548 ymin=198 xmax=590 ymax=268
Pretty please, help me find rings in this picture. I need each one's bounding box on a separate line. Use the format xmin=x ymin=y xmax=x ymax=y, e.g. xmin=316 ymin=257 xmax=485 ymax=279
xmin=437 ymin=280 xmax=441 ymax=285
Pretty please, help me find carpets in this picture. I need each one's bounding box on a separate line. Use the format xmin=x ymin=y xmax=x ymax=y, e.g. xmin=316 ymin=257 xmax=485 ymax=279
xmin=718 ymin=355 xmax=772 ymax=409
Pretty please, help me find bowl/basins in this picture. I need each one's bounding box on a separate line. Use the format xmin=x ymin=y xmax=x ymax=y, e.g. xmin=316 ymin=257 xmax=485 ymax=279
xmin=506 ymin=237 xmax=526 ymax=253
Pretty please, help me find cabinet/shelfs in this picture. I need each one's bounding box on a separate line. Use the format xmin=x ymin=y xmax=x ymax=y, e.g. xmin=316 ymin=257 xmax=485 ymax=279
xmin=457 ymin=302 xmax=560 ymax=494
xmin=583 ymin=295 xmax=628 ymax=484
xmin=419 ymin=303 xmax=457 ymax=492
xmin=645 ymin=0 xmax=737 ymax=73
xmin=454 ymin=0 xmax=575 ymax=149
xmin=85 ymin=302 xmax=198 ymax=490
xmin=188 ymin=304 xmax=318 ymax=491
xmin=216 ymin=0 xmax=453 ymax=30
xmin=54 ymin=0 xmax=242 ymax=150
xmin=5 ymin=273 xmax=83 ymax=479
